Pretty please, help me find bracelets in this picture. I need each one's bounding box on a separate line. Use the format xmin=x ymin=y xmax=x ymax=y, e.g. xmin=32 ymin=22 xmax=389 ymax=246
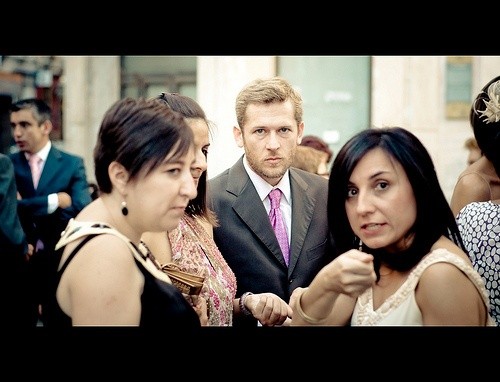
xmin=296 ymin=288 xmax=328 ymax=323
xmin=239 ymin=292 xmax=254 ymax=318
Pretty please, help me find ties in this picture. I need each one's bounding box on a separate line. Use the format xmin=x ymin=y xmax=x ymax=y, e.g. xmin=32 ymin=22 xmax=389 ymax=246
xmin=28 ymin=155 xmax=44 ymax=250
xmin=267 ymin=188 xmax=290 ymax=269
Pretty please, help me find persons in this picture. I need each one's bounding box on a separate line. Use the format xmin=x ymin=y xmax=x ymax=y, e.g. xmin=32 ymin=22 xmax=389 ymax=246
xmin=42 ymin=97 xmax=203 ymax=326
xmin=295 ymin=135 xmax=332 ymax=179
xmin=292 ymin=127 xmax=497 ymax=326
xmin=141 ymin=92 xmax=293 ymax=326
xmin=0 ymin=98 xmax=91 ymax=326
xmin=450 ymin=75 xmax=500 ymax=326
xmin=207 ymin=78 xmax=329 ymax=326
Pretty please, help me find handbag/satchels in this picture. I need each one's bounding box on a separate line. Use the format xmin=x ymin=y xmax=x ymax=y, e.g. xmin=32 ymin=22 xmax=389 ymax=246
xmin=159 ymin=261 xmax=205 ymax=309
